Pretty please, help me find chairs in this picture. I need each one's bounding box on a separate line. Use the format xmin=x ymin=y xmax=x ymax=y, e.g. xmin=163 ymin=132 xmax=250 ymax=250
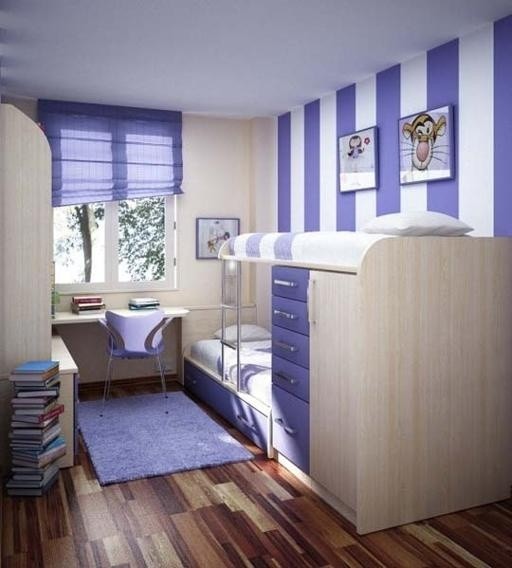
xmin=97 ymin=309 xmax=174 ymax=406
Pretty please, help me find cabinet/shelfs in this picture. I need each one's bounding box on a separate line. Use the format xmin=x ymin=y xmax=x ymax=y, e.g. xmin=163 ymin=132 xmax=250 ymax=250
xmin=0 ymin=104 xmax=79 ymax=475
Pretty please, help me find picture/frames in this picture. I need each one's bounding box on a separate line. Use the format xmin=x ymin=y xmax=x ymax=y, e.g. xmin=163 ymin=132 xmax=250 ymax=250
xmin=337 ymin=125 xmax=379 ymax=193
xmin=196 ymin=217 xmax=241 ymax=259
xmin=398 ymin=103 xmax=456 ymax=183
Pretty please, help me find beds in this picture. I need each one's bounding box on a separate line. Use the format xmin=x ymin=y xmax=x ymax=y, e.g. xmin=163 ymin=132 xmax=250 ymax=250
xmin=183 ymin=231 xmax=511 ymax=536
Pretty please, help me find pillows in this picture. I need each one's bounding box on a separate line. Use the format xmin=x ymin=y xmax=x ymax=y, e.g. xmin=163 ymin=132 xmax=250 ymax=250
xmin=364 ymin=210 xmax=478 ymax=237
xmin=214 ymin=324 xmax=272 ymax=344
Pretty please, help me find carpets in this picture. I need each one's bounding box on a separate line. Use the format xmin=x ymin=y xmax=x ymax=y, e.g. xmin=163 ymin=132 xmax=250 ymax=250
xmin=76 ymin=389 xmax=256 ymax=487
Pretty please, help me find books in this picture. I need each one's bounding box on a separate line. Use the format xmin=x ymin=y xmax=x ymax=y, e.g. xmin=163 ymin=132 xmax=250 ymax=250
xmin=127 ymin=298 xmax=161 ymax=311
xmin=4 ymin=362 xmax=67 ymax=497
xmin=71 ymin=295 xmax=107 ymax=315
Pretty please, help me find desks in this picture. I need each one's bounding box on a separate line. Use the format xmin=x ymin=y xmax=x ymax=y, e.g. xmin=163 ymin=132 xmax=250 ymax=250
xmin=52 ymin=307 xmax=190 ymax=386
